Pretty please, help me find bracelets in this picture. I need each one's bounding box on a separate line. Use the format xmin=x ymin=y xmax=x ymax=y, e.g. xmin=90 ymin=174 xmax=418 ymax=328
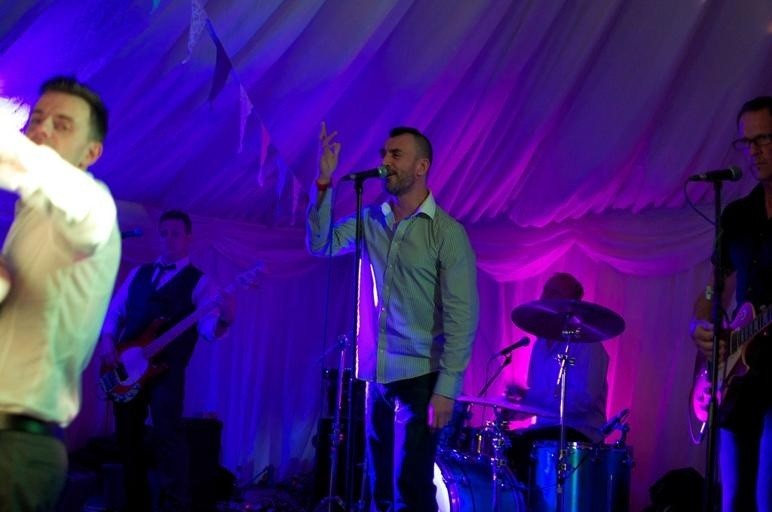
xmin=218 ymin=315 xmax=236 ymax=327
xmin=316 ymin=180 xmax=330 ymax=193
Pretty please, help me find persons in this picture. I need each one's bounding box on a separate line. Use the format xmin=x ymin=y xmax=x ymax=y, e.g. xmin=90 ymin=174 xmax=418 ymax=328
xmin=299 ymin=118 xmax=485 ymax=509
xmin=0 ymin=74 xmax=123 ymax=511
xmin=499 ymin=269 xmax=611 ymax=511
xmin=93 ymin=205 xmax=239 ymax=493
xmin=688 ymin=94 xmax=771 ymax=509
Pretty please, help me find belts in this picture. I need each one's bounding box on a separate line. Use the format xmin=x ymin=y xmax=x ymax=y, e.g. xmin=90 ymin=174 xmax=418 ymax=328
xmin=1 ymin=414 xmax=69 ymax=445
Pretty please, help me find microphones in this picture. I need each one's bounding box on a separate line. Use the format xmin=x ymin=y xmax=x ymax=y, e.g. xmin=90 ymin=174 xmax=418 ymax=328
xmin=121 ymin=227 xmax=145 ymax=240
xmin=601 ymin=409 xmax=627 ymax=435
xmin=500 ymin=337 xmax=529 ymax=354
xmin=342 ymin=166 xmax=388 ymax=185
xmin=689 ymin=166 xmax=743 ymax=183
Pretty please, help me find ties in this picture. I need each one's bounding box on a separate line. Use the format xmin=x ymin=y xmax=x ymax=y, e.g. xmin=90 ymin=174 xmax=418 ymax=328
xmin=152 ymin=265 xmax=176 ymax=291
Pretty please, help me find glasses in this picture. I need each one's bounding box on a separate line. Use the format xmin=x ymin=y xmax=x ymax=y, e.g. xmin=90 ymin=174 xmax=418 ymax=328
xmin=732 ymin=134 xmax=772 ymax=149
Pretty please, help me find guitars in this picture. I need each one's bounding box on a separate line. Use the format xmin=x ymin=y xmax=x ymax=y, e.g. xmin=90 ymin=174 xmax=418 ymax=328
xmin=99 ymin=259 xmax=271 ymax=403
xmin=689 ymin=301 xmax=772 ymax=421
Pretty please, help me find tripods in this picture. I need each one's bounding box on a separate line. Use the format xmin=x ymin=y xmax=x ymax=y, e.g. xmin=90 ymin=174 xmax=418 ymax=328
xmin=314 ymin=331 xmax=354 ymax=511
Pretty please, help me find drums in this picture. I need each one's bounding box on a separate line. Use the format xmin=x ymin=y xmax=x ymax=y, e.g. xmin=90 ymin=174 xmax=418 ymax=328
xmin=474 ymin=429 xmax=514 ymax=461
xmin=527 ymin=441 xmax=636 ymax=512
xmin=433 ymin=448 xmax=527 ymax=512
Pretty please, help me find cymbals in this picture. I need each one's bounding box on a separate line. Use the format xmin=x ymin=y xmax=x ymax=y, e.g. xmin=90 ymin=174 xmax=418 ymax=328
xmin=512 ymin=300 xmax=624 ymax=343
xmin=460 ymin=396 xmax=560 ymax=419
